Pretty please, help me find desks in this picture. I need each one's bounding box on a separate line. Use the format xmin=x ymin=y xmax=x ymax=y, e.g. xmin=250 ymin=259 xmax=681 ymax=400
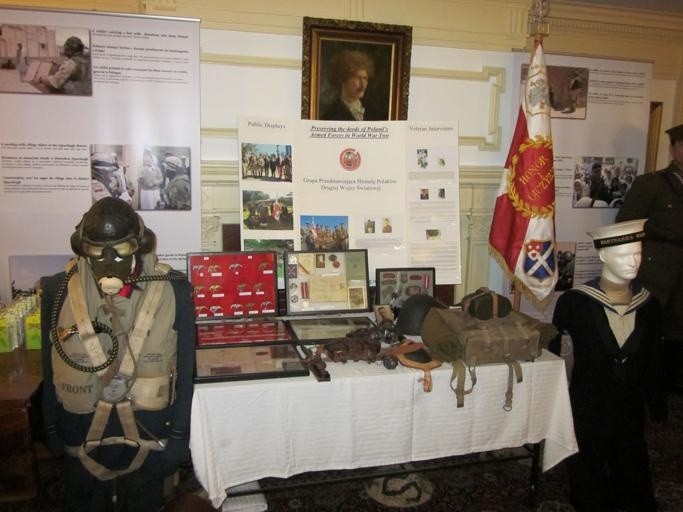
xmin=0 ymin=348 xmax=44 ymax=511
xmin=189 ymin=304 xmax=564 ymax=511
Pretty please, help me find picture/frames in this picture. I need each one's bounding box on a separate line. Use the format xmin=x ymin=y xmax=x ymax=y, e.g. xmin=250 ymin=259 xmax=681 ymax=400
xmin=299 ymin=15 xmax=412 ymax=121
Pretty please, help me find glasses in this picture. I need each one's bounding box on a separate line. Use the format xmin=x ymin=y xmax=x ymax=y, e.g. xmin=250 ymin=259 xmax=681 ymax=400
xmin=81 ymin=234 xmax=140 ymax=259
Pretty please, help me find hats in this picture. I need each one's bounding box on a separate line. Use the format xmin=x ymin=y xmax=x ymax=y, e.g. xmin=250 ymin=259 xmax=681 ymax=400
xmin=90 ymin=153 xmax=119 ymax=172
xmin=665 ymin=124 xmax=683 ymax=146
xmin=161 ymin=152 xmax=182 ymax=172
xmin=587 ymin=218 xmax=647 ymax=248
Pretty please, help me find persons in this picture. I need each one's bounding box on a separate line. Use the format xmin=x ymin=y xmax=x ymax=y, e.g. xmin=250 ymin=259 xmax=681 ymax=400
xmin=37 ymin=35 xmax=92 ymax=94
xmin=304 ymin=222 xmax=349 ymax=251
xmin=90 ymin=150 xmax=192 ymax=210
xmin=321 ymin=50 xmax=379 ymax=120
xmin=420 ymin=189 xmax=428 ymax=200
xmin=567 ymin=71 xmax=583 ymax=113
xmin=574 ymin=159 xmax=636 ymax=207
xmin=549 ymin=218 xmax=655 ymax=498
xmin=382 ymin=219 xmax=392 ymax=234
xmin=316 ymin=254 xmax=325 ymax=268
xmin=14 ymin=42 xmax=29 ymax=84
xmin=242 ymin=151 xmax=291 ymax=181
xmin=614 ymin=125 xmax=683 ymax=428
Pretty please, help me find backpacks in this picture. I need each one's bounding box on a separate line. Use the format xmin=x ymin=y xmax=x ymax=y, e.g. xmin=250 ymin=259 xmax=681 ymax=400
xmin=420 ymin=287 xmax=558 ymax=366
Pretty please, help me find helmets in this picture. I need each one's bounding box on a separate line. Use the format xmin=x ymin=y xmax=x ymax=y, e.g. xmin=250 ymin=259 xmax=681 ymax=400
xmin=396 ymin=294 xmax=444 ymax=336
xmin=70 ymin=197 xmax=156 ymax=256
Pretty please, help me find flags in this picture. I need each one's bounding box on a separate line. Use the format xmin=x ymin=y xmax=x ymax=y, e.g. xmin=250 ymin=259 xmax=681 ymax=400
xmin=490 ymin=40 xmax=561 ymax=313
